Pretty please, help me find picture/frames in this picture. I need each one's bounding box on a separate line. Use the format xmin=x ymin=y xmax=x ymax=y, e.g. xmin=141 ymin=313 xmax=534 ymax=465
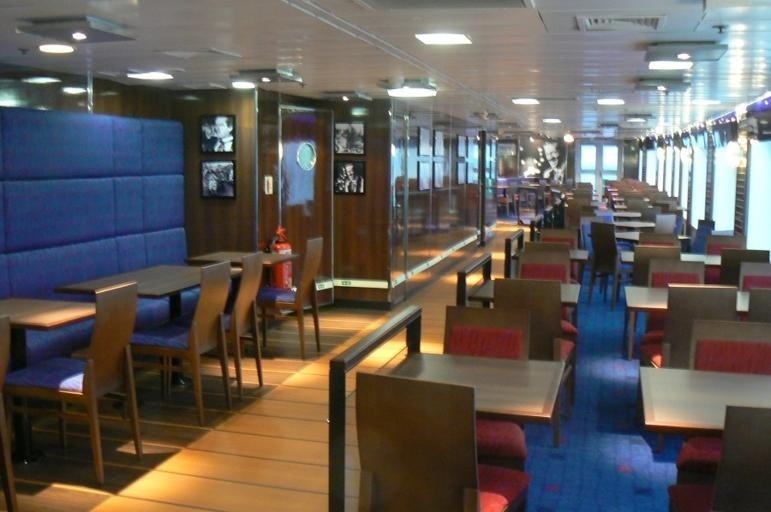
xmin=334 ymin=120 xmax=367 ymax=157
xmin=334 ymin=160 xmax=367 ymax=196
xmin=516 ymin=135 xmax=569 ymax=180
xmin=197 ymin=112 xmax=238 ymax=155
xmin=199 ymin=158 xmax=239 ymax=201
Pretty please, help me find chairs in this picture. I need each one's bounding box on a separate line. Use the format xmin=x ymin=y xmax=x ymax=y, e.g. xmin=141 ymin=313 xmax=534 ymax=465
xmin=356 ymin=371 xmax=531 ymax=512
xmin=442 ymin=305 xmax=531 ymax=472
xmin=55 ymin=234 xmax=323 ymax=426
xmin=667 ymin=319 xmax=771 ymax=512
xmin=601 ymin=180 xmax=771 ymax=344
xmin=587 ymin=222 xmax=639 ymax=310
xmin=2 ymin=280 xmax=143 ymax=490
xmin=0 ymin=315 xmax=19 ymax=512
xmin=515 ymin=182 xmax=598 ymax=283
xmin=493 ymin=277 xmax=575 ymax=408
xmin=639 ymin=283 xmax=737 ymax=453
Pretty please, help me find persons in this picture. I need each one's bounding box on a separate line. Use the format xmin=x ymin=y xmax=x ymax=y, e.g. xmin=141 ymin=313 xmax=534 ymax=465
xmin=200 ymin=115 xmax=233 ymax=153
xmin=541 ymin=139 xmax=565 ymax=184
xmin=344 ymin=163 xmax=364 ymax=193
xmin=341 ymin=124 xmax=356 ymax=149
xmin=205 ymin=164 xmax=235 ymax=195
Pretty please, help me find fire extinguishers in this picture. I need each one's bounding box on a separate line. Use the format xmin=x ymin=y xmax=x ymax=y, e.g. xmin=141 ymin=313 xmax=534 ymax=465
xmin=270 ymin=223 xmax=293 ymax=289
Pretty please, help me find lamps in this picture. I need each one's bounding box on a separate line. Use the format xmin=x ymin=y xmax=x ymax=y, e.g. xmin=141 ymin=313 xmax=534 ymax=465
xmin=644 ymin=40 xmax=730 ymax=65
xmin=16 ymin=16 xmax=140 ymax=48
xmin=238 ymin=65 xmax=306 ymax=86
xmin=621 ymin=113 xmax=653 ymax=124
xmin=631 ymin=78 xmax=693 ymax=95
xmin=384 ymin=77 xmax=439 ymax=99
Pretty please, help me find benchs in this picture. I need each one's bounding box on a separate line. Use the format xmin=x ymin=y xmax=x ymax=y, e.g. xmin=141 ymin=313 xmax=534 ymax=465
xmin=24 ymin=318 xmax=94 ymax=359
